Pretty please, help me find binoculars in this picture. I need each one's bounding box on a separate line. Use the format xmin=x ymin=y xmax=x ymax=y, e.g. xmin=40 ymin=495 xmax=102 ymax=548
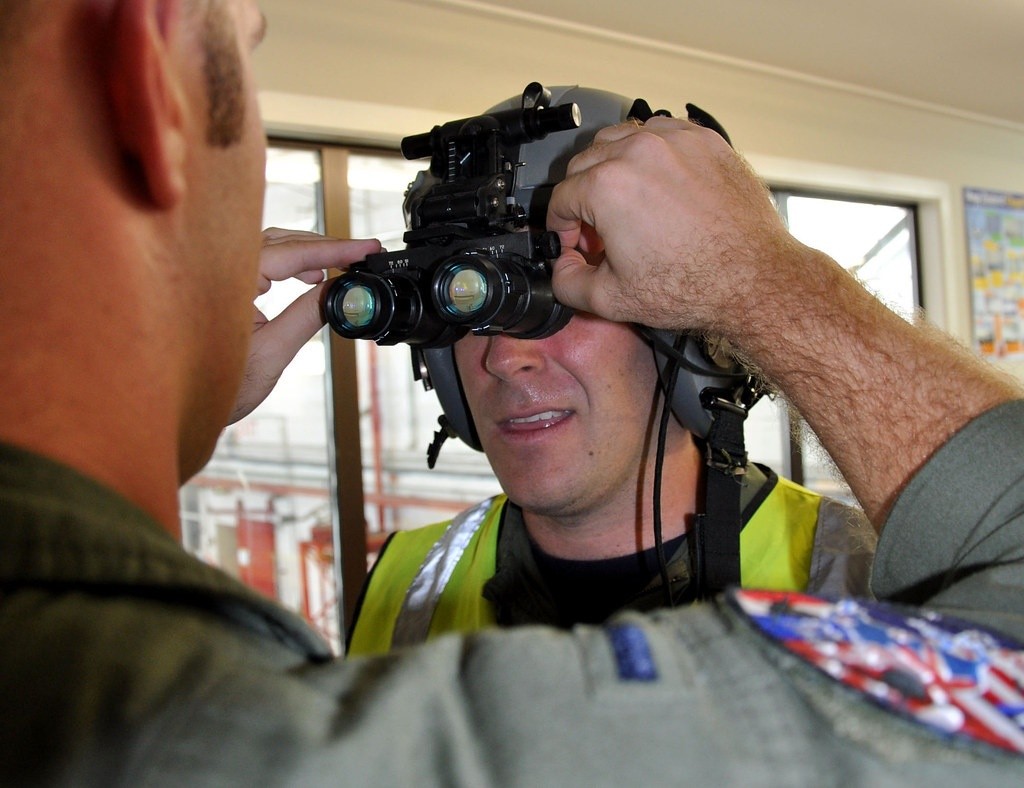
xmin=316 ymin=79 xmax=583 ymax=341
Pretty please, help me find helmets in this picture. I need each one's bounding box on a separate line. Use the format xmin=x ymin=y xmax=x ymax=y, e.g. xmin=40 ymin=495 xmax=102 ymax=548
xmin=405 ymin=81 xmax=750 ymax=469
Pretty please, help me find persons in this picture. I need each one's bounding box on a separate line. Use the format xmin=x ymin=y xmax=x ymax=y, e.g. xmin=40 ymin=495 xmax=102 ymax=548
xmin=325 ymin=84 xmax=881 ymax=661
xmin=0 ymin=0 xmax=1024 ymax=788
